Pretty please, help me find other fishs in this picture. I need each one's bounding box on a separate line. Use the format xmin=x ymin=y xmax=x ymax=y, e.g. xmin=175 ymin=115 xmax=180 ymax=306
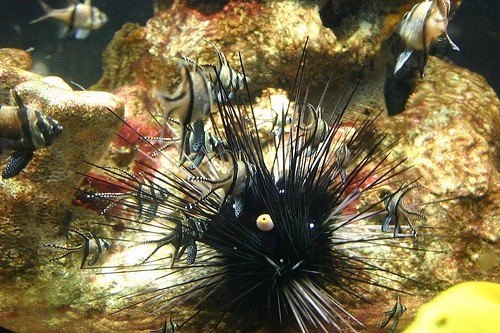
xmin=29 ymin=0 xmax=107 ymax=38
xmin=1 ymin=88 xmax=64 ymax=180
xmin=392 ymin=0 xmax=463 ymax=79
xmin=44 ymin=39 xmax=429 ymax=333
